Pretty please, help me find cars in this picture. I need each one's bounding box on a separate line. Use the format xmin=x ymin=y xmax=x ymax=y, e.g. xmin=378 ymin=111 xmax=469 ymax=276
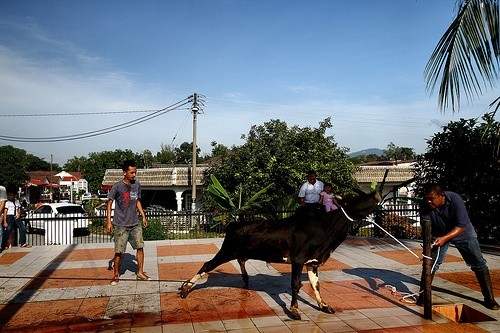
xmin=22 ymin=203 xmax=89 ymax=234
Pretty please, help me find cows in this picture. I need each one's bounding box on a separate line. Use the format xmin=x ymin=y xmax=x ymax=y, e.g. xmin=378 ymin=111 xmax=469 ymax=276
xmin=178 ymin=189 xmax=382 ymax=320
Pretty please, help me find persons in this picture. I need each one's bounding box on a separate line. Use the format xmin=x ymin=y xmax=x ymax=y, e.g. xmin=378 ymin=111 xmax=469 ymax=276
xmin=299 ymin=170 xmax=338 ymax=212
xmin=0 ymin=183 xmax=28 ymax=254
xmin=417 ymin=182 xmax=500 ymax=310
xmin=106 ymin=160 xmax=153 ymax=286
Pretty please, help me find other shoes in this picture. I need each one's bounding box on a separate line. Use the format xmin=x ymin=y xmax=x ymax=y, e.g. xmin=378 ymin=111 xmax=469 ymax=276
xmin=0 ymin=248 xmax=2 ymax=254
xmin=109 ymin=276 xmax=120 ymax=286
xmin=21 ymin=243 xmax=32 ymax=248
xmin=137 ymin=272 xmax=151 ymax=280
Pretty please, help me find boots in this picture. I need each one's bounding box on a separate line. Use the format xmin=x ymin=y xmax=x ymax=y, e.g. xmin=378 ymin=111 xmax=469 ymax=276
xmin=416 ymin=272 xmax=435 ymax=306
xmin=474 ymin=267 xmax=499 ymax=310
